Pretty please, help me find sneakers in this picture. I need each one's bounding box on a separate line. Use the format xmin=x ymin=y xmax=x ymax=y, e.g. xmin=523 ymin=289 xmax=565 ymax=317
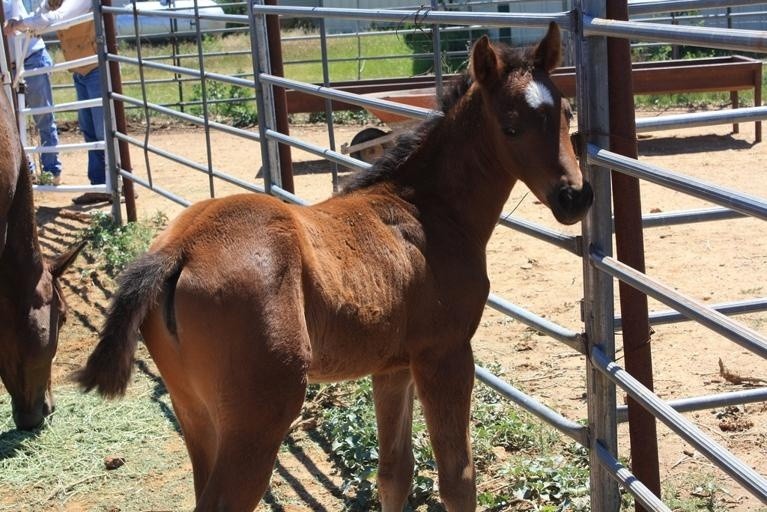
xmin=72 ymin=186 xmax=113 ymax=208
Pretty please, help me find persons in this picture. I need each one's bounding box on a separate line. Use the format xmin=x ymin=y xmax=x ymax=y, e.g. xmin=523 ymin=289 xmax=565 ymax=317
xmin=1 ymin=1 xmax=63 ymax=187
xmin=6 ymin=1 xmax=138 ymax=203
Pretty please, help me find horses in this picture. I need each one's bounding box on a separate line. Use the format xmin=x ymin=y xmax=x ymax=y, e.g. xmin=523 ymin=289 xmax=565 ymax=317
xmin=0 ymin=80 xmax=89 ymax=435
xmin=63 ymin=16 xmax=595 ymax=511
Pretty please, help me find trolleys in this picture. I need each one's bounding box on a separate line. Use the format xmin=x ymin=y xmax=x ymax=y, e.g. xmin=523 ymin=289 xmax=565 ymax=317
xmin=341 ymin=87 xmax=450 ymax=164
